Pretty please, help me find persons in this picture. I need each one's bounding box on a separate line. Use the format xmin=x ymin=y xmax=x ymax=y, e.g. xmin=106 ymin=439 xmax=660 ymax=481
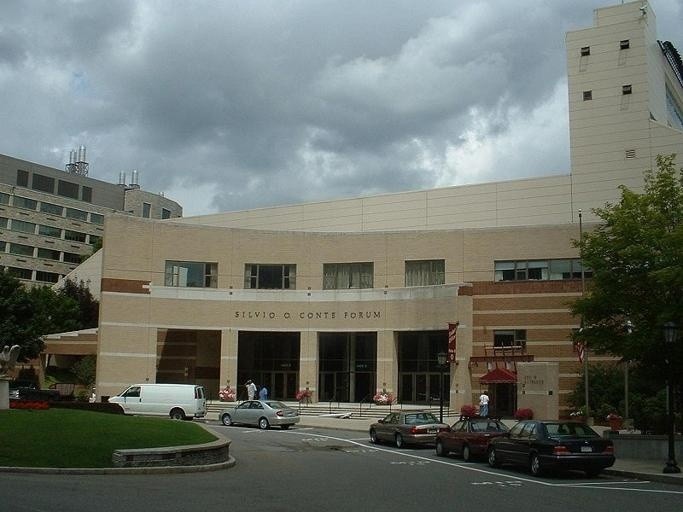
xmin=479 ymin=390 xmax=489 ymax=417
xmin=244 ymin=379 xmax=257 ymax=400
xmin=258 ymin=383 xmax=268 ymax=400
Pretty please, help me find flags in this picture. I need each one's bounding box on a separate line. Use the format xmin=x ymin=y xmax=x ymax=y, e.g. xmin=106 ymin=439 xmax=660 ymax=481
xmin=575 ymin=327 xmax=586 ymax=364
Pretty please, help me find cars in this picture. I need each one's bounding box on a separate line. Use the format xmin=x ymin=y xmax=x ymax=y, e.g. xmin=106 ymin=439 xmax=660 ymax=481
xmin=220 ymin=400 xmax=299 ymax=429
xmin=369 ymin=410 xmax=616 ymax=477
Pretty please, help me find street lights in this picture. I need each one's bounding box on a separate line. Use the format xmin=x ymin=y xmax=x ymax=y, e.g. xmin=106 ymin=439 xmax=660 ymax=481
xmin=663 ymin=322 xmax=680 ymax=473
xmin=437 ymin=353 xmax=448 ymax=423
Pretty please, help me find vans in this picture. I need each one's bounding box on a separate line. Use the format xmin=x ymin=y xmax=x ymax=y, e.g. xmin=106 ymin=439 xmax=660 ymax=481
xmin=108 ymin=384 xmax=207 ymax=420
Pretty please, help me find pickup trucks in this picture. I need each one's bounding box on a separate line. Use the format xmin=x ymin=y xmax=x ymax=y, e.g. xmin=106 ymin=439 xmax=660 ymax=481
xmin=0 ymin=375 xmax=60 ymax=401
